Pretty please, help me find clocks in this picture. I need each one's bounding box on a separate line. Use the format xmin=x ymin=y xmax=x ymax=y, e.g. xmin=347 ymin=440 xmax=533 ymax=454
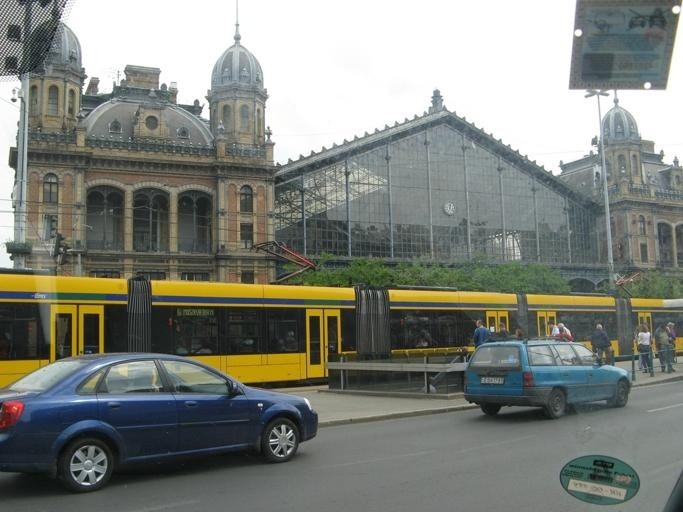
xmin=443 ymin=201 xmax=456 ymax=215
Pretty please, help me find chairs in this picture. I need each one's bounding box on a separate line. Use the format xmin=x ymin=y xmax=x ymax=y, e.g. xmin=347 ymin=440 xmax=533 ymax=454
xmin=131 ymin=368 xmax=157 ymax=393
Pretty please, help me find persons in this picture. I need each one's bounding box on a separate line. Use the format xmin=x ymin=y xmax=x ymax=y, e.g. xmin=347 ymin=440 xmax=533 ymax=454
xmin=635 ymin=322 xmax=677 ymax=377
xmin=474 ymin=319 xmax=492 ymax=346
xmin=549 ymin=321 xmax=574 ymax=341
xmin=591 ymin=323 xmax=612 ymax=365
xmin=515 ymin=324 xmax=525 ymax=340
xmin=176 ymin=330 xmax=298 ymax=356
xmin=391 ymin=326 xmax=433 ymax=349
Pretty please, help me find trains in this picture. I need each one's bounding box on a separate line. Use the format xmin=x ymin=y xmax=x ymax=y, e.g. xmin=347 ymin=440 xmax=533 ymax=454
xmin=0 ymin=273 xmax=683 ymax=388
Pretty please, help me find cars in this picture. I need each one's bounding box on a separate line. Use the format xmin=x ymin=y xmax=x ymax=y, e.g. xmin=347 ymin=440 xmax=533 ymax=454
xmin=0 ymin=351 xmax=319 ymax=494
xmin=464 ymin=339 xmax=633 ymax=419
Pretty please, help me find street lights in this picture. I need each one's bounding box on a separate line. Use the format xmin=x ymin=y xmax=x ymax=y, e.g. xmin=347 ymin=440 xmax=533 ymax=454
xmin=584 ymin=86 xmax=616 ymax=291
